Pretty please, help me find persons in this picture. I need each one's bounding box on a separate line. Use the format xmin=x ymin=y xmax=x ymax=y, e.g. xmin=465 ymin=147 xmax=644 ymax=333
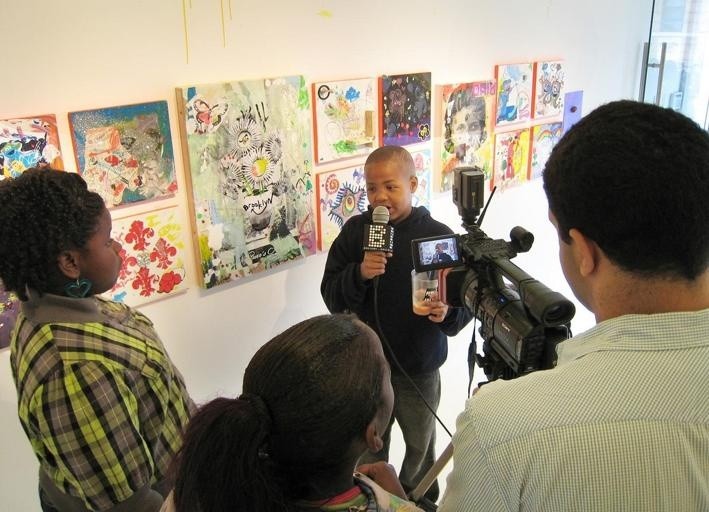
xmin=0 ymin=168 xmax=200 ymax=512
xmin=321 ymin=144 xmax=460 ymax=512
xmin=430 ymin=100 xmax=708 ymax=511
xmin=156 ymin=315 xmax=429 ymax=512
xmin=431 ymin=242 xmax=453 ymax=264
xmin=441 ymin=85 xmax=488 ymax=192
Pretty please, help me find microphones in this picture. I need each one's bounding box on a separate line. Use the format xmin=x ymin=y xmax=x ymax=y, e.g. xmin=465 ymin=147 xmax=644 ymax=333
xmin=363 ymin=206 xmax=395 ymax=289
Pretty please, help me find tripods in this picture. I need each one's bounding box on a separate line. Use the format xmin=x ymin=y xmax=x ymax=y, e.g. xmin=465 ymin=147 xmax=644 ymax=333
xmin=405 ymin=379 xmax=492 ymax=503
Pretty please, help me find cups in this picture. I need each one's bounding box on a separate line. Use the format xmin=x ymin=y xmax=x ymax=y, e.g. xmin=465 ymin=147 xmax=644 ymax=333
xmin=411 ymin=269 xmax=438 ymax=316
